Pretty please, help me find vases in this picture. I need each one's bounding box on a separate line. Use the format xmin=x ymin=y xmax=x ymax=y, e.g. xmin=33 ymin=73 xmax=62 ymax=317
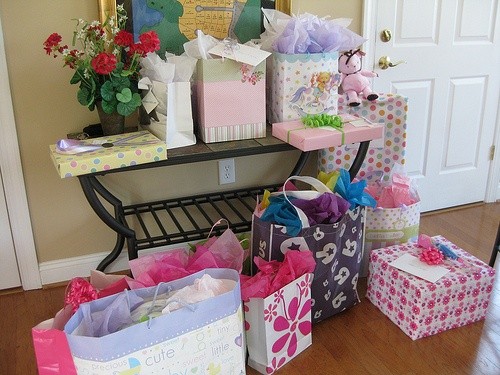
xmin=94 ymin=94 xmax=139 ymax=137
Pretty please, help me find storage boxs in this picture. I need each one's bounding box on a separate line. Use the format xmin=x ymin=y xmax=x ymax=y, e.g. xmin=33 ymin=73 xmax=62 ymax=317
xmin=48 ymin=130 xmax=168 ymax=179
xmin=271 ymin=113 xmax=385 ymax=152
xmin=364 ymin=234 xmax=498 ymax=342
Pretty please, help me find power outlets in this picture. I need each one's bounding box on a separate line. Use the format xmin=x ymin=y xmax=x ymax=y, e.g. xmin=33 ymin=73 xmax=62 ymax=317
xmin=218 ymin=157 xmax=235 ymax=185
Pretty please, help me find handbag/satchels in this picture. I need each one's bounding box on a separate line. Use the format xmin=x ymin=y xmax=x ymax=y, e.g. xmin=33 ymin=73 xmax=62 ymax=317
xmin=242 ymin=271 xmax=313 ymax=375
xmin=194 ymin=56 xmax=267 ymax=143
xmin=138 ymin=79 xmax=197 ymax=151
xmin=32 ymin=269 xmax=132 ymax=375
xmin=360 ymin=184 xmax=421 ymax=278
xmin=63 ymin=268 xmax=248 ymax=375
xmin=268 ymin=48 xmax=340 ymax=123
xmin=251 ymin=175 xmax=361 ymax=327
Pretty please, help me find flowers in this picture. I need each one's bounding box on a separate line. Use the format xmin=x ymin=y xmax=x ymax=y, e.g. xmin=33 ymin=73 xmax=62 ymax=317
xmin=42 ymin=3 xmax=161 ymax=116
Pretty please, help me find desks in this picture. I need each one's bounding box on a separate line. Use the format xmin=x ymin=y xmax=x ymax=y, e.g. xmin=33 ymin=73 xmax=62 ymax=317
xmin=64 ymin=115 xmax=373 ymax=279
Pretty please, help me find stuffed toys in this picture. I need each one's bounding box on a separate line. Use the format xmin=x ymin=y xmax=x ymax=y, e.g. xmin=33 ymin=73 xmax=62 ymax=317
xmin=338 ymin=49 xmax=378 ymax=107
